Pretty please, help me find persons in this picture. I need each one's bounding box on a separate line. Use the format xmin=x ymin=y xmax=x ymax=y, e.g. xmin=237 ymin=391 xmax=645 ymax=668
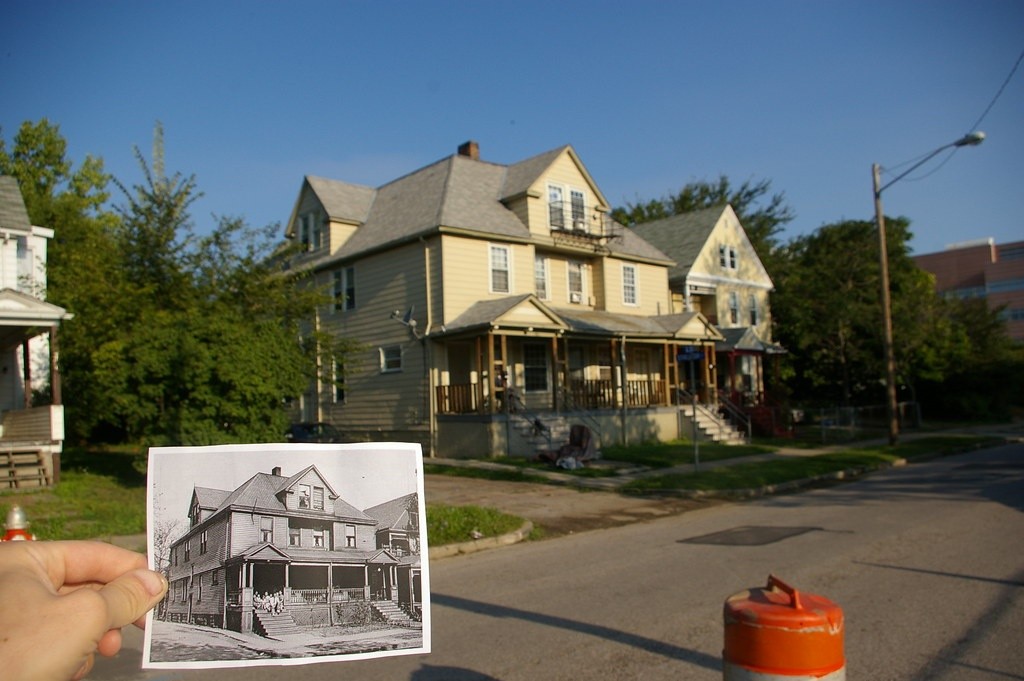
xmin=0 ymin=540 xmax=168 ymax=681
xmin=494 ymin=371 xmax=515 ymax=413
xmin=253 ymin=591 xmax=284 ymax=617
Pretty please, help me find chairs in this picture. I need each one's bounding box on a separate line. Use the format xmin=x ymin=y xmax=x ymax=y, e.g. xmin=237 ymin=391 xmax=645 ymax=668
xmin=539 ymin=424 xmax=596 ymax=468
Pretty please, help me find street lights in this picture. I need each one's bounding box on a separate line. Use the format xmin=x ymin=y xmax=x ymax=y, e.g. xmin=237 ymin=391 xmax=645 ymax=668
xmin=872 ymin=129 xmax=985 ymax=447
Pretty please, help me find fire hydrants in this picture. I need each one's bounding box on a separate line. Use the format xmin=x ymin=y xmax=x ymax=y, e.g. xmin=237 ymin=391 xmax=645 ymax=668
xmin=0 ymin=505 xmax=37 ymax=541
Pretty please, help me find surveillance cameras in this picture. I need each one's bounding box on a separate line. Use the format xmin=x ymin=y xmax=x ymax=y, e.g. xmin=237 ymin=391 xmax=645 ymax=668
xmin=390 ymin=309 xmax=400 ymax=320
